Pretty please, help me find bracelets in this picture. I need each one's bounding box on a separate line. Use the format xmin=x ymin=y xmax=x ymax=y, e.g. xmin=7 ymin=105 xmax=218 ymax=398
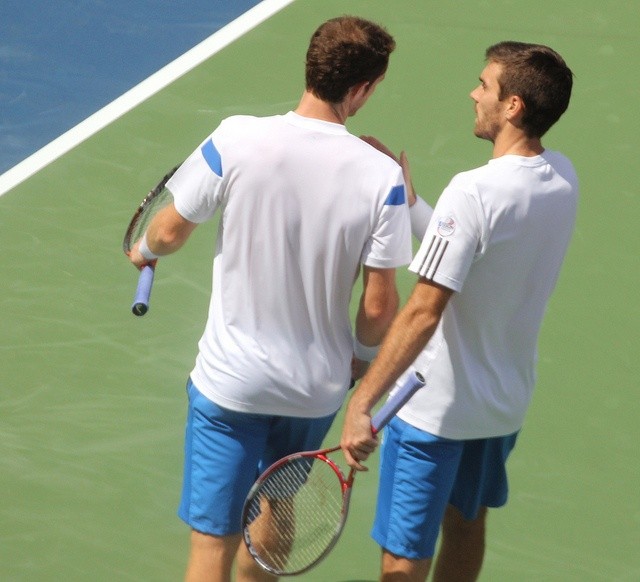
xmin=354 ymin=338 xmax=383 ymax=362
xmin=409 ymin=194 xmax=435 ymax=242
xmin=138 ymin=232 xmax=160 ymax=260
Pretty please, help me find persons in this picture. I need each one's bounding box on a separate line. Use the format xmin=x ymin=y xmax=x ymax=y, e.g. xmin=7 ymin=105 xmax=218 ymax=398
xmin=341 ymin=40 xmax=579 ymax=581
xmin=130 ymin=16 xmax=411 ymax=581
